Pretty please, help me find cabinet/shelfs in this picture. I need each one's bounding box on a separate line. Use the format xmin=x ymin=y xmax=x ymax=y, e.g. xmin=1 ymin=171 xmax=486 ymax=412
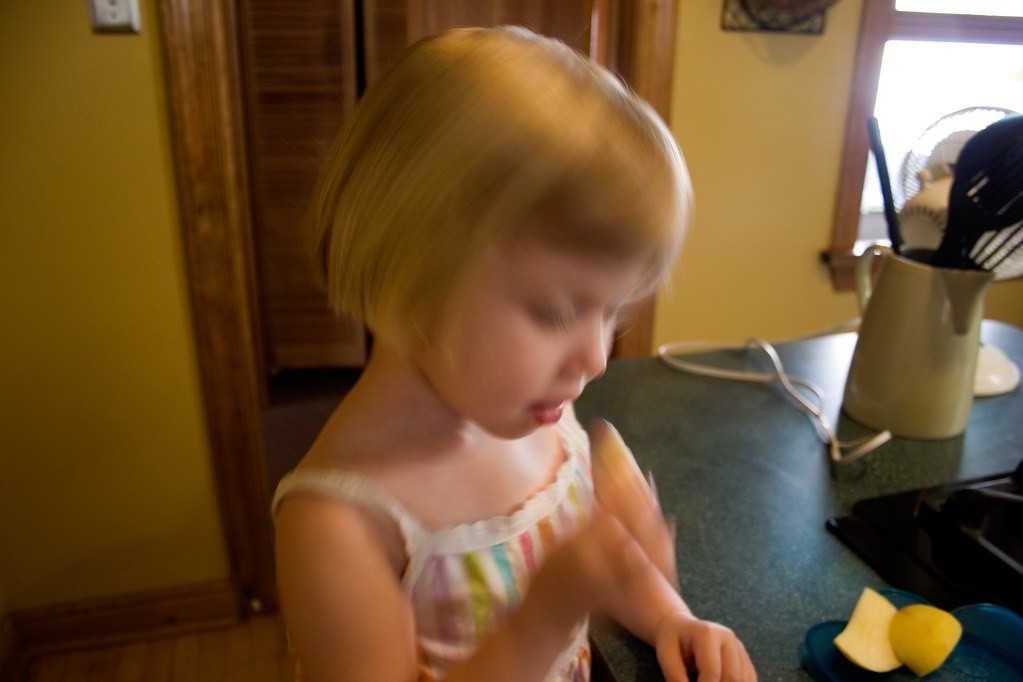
xmin=233 ymin=0 xmax=616 ymax=376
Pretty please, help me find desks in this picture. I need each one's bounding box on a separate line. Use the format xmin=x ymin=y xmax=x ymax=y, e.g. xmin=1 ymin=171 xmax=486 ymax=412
xmin=573 ymin=312 xmax=1023 ymax=682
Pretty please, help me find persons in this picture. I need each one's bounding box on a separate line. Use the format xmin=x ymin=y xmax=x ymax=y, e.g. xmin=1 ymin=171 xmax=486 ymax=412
xmin=270 ymin=23 xmax=756 ymax=682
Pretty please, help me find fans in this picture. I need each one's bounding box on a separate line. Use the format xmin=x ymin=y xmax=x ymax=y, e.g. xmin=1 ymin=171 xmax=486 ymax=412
xmin=894 ymin=104 xmax=1023 ymax=398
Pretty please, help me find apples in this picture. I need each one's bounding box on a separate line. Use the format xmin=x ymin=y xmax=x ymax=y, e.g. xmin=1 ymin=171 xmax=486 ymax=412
xmin=834 ymin=587 xmax=904 ymax=672
xmin=890 ymin=604 xmax=962 ymax=677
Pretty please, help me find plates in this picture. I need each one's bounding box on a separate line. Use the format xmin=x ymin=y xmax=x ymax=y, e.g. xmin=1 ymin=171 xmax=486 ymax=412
xmin=806 ymin=590 xmax=1023 ymax=682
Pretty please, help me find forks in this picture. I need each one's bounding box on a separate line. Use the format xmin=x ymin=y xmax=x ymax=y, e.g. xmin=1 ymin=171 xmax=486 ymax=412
xmin=966 ymin=219 xmax=1023 ymax=272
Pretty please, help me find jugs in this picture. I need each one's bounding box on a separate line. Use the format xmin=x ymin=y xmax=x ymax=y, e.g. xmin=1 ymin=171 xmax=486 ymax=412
xmin=841 ymin=244 xmax=990 ymax=440
